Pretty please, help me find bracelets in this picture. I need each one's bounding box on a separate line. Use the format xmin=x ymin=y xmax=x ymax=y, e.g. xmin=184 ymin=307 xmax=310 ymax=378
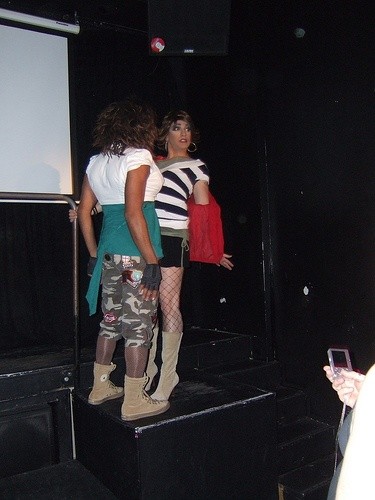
xmin=92 ymin=206 xmax=99 ymax=214
xmin=92 ymin=207 xmax=96 ymax=215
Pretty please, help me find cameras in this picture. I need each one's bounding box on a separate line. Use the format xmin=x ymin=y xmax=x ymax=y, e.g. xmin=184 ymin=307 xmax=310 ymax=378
xmin=328 ymin=348 xmax=352 ymax=373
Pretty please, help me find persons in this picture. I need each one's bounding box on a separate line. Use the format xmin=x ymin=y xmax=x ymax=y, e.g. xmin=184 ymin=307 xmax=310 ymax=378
xmin=69 ymin=110 xmax=234 ymax=401
xmin=323 ymin=363 xmax=375 ymax=500
xmin=77 ymin=101 xmax=171 ymax=421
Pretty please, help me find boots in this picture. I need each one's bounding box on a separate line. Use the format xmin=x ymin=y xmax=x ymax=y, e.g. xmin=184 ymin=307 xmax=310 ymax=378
xmin=88 ymin=361 xmax=124 ymax=405
xmin=150 ymin=331 xmax=183 ymax=401
xmin=144 ymin=328 xmax=158 ymax=391
xmin=121 ymin=372 xmax=170 ymax=421
